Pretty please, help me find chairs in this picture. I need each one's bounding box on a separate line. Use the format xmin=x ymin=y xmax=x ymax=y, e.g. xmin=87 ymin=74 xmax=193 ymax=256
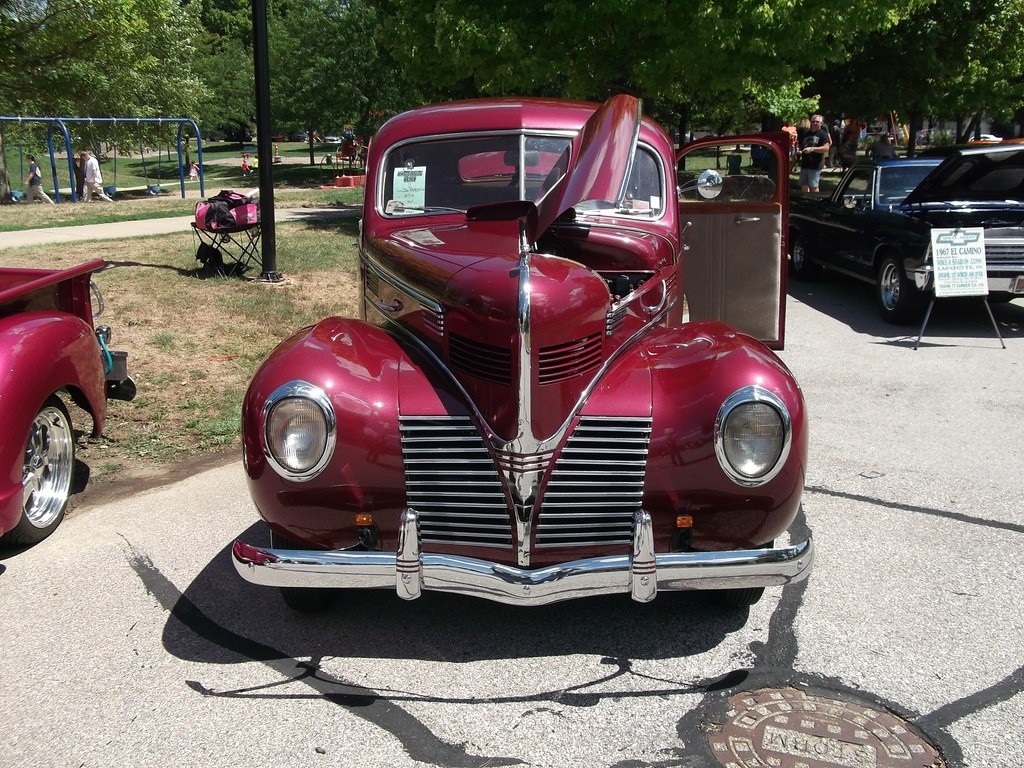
xmin=187 ymin=219 xmax=263 ymax=276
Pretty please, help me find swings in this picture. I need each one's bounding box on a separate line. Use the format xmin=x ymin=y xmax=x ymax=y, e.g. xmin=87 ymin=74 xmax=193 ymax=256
xmin=135 ymin=121 xmax=161 ymax=195
xmin=88 ymin=119 xmax=117 ymax=199
xmin=0 ymin=119 xmax=25 ymax=202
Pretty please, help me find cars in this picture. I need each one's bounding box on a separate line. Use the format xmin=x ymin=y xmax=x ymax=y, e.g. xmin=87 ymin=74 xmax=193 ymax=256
xmin=969 ymin=134 xmax=1004 ymax=141
xmin=673 ymin=132 xmax=698 ymax=145
xmin=789 ymin=139 xmax=1023 ymax=324
xmin=0 ymin=252 xmax=142 ymax=560
xmin=227 ymin=94 xmax=819 ymax=607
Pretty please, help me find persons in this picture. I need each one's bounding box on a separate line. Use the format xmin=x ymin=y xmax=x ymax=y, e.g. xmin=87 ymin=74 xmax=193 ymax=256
xmin=726 ymin=112 xmax=898 ymax=194
xmin=189 ymin=160 xmax=200 ymax=182
xmin=80 ymin=151 xmax=115 ymax=203
xmin=241 ymin=151 xmax=259 ymax=179
xmin=24 ymin=153 xmax=55 ymax=204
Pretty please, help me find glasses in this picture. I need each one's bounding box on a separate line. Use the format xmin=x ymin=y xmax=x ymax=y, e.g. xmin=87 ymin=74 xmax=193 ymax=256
xmin=812 ymin=121 xmax=821 ymax=123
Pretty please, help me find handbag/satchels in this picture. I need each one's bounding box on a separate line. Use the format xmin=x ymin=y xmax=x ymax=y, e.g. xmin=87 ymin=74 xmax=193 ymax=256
xmin=195 ymin=189 xmax=257 ymax=231
